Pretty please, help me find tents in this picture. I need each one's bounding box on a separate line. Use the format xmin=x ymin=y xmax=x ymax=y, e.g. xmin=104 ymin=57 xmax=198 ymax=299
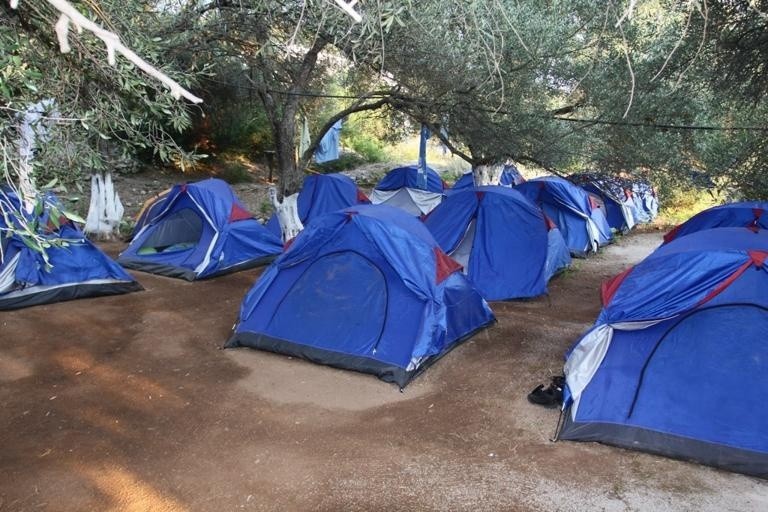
xmin=116 ymin=178 xmax=285 ymax=282
xmin=0 ymin=183 xmax=147 ymax=313
xmin=227 ymin=158 xmax=660 ymax=393
xmin=552 ymin=201 xmax=767 ymax=481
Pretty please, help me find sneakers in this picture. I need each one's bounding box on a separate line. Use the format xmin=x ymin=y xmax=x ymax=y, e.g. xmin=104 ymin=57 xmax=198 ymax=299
xmin=528 ymin=376 xmax=563 ymax=409
